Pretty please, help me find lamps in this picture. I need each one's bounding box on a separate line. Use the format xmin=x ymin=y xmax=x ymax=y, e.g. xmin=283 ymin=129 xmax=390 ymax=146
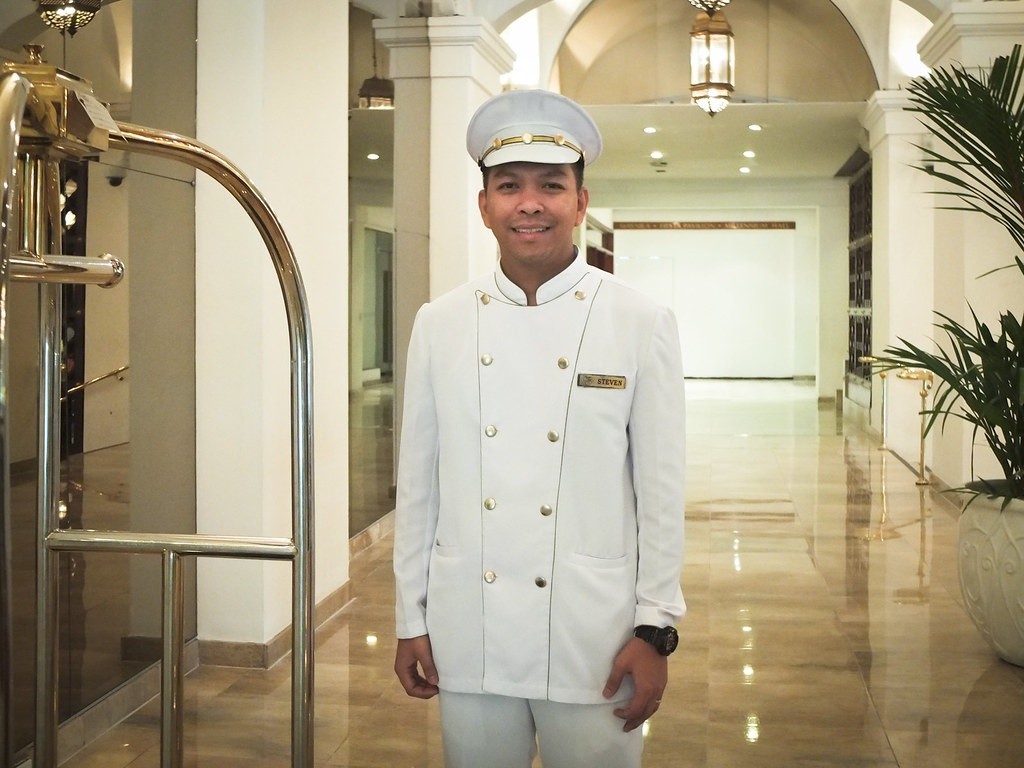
xmin=357 ymin=29 xmax=393 ymax=111
xmin=39 ymin=0 xmax=102 ymax=38
xmin=688 ymin=0 xmax=735 ymax=117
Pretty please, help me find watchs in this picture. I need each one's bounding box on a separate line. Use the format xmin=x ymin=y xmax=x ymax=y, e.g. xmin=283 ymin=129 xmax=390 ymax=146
xmin=632 ymin=625 xmax=678 ymax=656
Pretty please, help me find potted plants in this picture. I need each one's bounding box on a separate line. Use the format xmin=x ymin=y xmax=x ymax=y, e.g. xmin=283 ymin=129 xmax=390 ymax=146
xmin=869 ymin=43 xmax=1024 ymax=667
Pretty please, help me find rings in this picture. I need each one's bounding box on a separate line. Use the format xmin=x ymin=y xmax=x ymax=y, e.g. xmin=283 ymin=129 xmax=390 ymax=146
xmin=656 ymin=700 xmax=661 ymax=703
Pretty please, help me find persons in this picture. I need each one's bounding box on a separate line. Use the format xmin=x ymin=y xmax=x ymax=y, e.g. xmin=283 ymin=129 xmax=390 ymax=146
xmin=393 ymin=89 xmax=688 ymax=768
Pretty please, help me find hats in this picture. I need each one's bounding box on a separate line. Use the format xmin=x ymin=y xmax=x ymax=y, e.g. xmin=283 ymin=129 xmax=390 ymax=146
xmin=466 ymin=90 xmax=603 ymax=170
